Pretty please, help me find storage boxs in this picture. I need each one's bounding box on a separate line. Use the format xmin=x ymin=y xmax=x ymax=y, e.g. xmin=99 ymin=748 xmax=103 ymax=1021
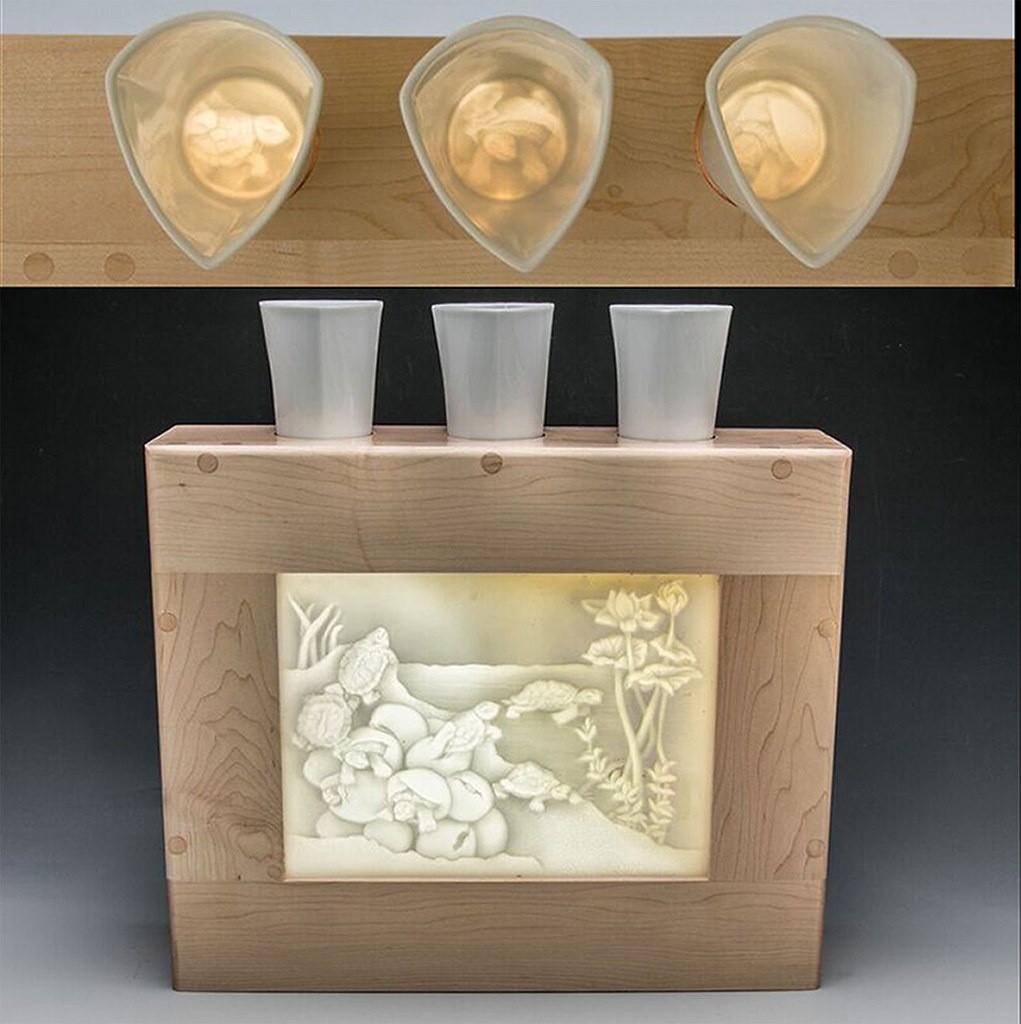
xmin=143 ymin=427 xmax=851 ymax=995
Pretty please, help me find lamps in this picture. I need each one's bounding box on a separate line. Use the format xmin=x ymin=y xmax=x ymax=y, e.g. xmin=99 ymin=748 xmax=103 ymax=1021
xmin=696 ymin=14 xmax=921 ymax=271
xmin=397 ymin=13 xmax=615 ymax=272
xmin=105 ymin=7 xmax=326 ymax=273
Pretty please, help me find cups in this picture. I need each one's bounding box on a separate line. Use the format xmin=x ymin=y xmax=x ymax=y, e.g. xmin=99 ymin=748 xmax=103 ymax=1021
xmin=105 ymin=10 xmax=324 ymax=271
xmin=691 ymin=15 xmax=919 ymax=270
xmin=397 ymin=15 xmax=614 ymax=274
xmin=609 ymin=305 xmax=733 ymax=442
xmin=260 ymin=300 xmax=383 ymax=439
xmin=432 ymin=303 xmax=554 ymax=440
